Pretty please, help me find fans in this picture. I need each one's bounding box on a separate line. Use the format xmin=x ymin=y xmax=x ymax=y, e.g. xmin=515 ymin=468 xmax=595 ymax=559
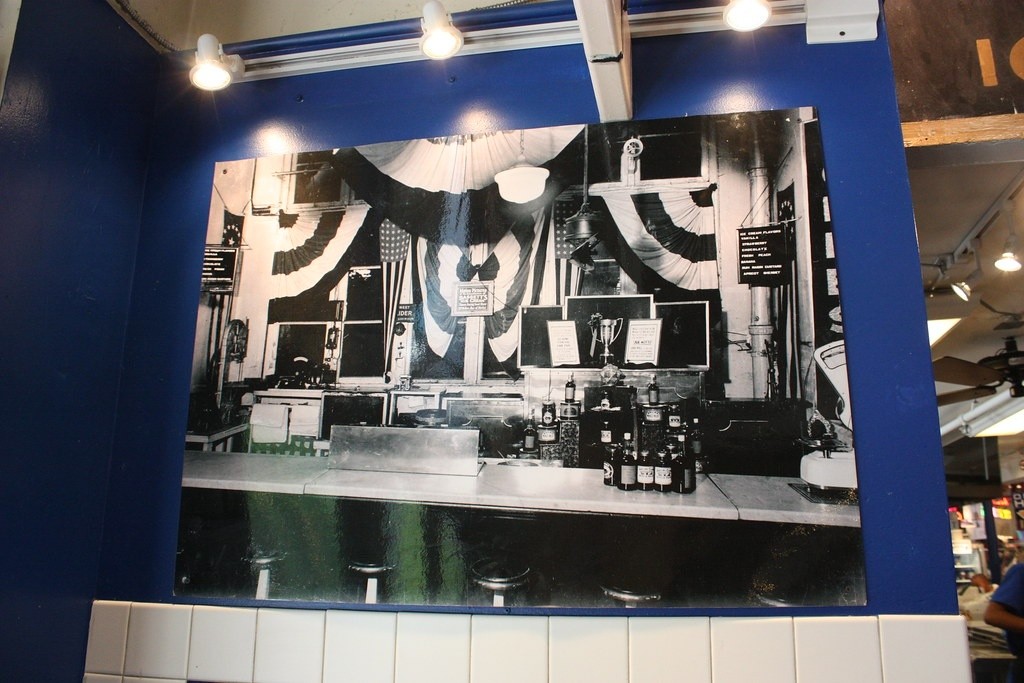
xmin=930 ymin=340 xmax=1024 ymax=405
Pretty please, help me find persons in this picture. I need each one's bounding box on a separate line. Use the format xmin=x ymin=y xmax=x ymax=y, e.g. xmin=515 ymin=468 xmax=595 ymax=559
xmin=970 ymin=565 xmax=1024 ymax=683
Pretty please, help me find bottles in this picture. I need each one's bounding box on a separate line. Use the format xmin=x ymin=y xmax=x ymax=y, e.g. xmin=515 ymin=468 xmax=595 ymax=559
xmin=522 ymin=374 xmax=707 ymax=494
xmin=292 ymin=363 xmax=332 ymax=388
xmin=768 ymin=360 xmax=783 ymax=399
xmin=948 ymin=507 xmax=989 ymax=596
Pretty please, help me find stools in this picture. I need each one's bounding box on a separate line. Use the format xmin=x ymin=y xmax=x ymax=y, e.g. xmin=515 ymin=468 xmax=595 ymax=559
xmin=752 ymin=578 xmax=819 ymax=607
xmin=242 ymin=549 xmax=287 ymax=600
xmin=600 ymin=581 xmax=661 ymax=608
xmin=471 ymin=557 xmax=529 ymax=607
xmin=348 ymin=548 xmax=398 ymax=605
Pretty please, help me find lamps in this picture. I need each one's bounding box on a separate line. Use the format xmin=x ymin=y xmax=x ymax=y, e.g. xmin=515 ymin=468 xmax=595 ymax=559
xmin=495 ymin=131 xmax=550 ymax=205
xmin=419 ymin=1 xmax=464 ymax=61
xmin=950 ymin=238 xmax=986 ymax=302
xmin=994 ymin=200 xmax=1022 ymax=272
xmin=189 ymin=34 xmax=244 ymax=91
xmin=722 ymin=0 xmax=772 ymax=32
xmin=566 ymin=124 xmax=604 ymax=272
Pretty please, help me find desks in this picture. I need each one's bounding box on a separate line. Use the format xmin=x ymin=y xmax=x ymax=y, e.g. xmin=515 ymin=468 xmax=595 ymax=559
xmin=181 ymin=450 xmax=861 ymax=527
xmin=702 ymin=397 xmax=813 ymax=478
xmin=388 ymin=388 xmax=446 ymax=425
xmin=185 ymin=425 xmax=247 ymax=451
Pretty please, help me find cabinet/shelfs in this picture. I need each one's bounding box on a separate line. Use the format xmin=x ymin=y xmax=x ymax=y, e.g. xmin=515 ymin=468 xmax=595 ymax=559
xmin=954 ymin=565 xmax=979 ymax=584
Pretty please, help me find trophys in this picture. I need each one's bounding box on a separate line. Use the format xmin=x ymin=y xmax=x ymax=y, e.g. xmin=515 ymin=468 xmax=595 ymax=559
xmin=590 ymin=314 xmax=623 ymax=365
